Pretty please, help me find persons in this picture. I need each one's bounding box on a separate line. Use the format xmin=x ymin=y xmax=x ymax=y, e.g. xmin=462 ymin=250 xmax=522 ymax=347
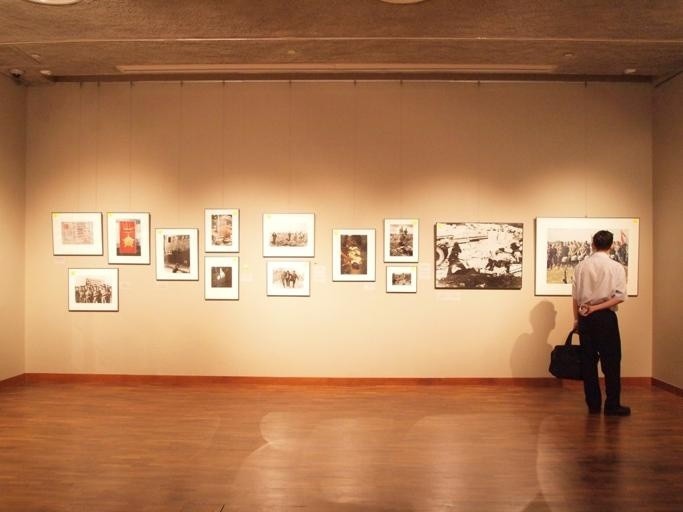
xmin=75 ymin=279 xmax=111 ymax=303
xmin=281 ymin=271 xmax=299 ymax=288
xmin=547 ymin=241 xmax=628 ymax=270
xmin=272 ymin=232 xmax=305 ymax=246
xmin=572 ymin=230 xmax=631 ymax=416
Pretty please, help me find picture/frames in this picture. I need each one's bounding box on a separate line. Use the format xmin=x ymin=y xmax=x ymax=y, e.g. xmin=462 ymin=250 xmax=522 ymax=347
xmin=107 ymin=211 xmax=151 ymax=265
xmin=67 ymin=267 xmax=119 ymax=312
xmin=52 ymin=212 xmax=103 ymax=255
xmin=536 ymin=217 xmax=638 ymax=296
xmin=204 ymin=208 xmax=240 ymax=253
xmin=333 ymin=229 xmax=375 ymax=282
xmin=386 ymin=265 xmax=417 ymax=292
xmin=384 ymin=219 xmax=418 ymax=262
xmin=155 ymin=227 xmax=200 ymax=281
xmin=203 ymin=256 xmax=240 ymax=301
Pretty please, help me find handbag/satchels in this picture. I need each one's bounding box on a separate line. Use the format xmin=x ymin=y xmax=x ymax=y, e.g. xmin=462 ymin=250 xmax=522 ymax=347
xmin=549 ymin=330 xmax=600 ymax=379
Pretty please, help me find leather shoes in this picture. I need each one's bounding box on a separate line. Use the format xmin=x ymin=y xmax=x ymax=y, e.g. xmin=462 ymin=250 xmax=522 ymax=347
xmin=604 ymin=406 xmax=630 ymax=415
